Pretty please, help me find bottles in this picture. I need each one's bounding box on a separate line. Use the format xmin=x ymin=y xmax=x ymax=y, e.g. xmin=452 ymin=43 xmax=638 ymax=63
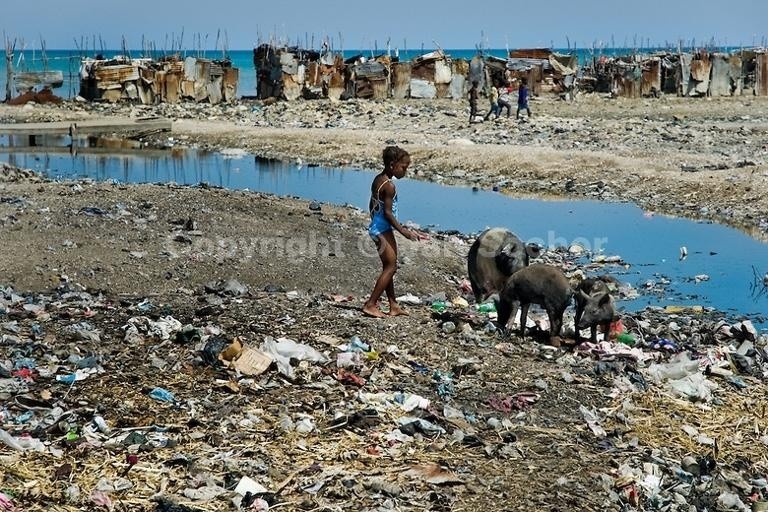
xmin=617 ymin=332 xmax=637 ymax=345
xmin=432 ymin=300 xmax=445 ymax=311
xmin=478 ymin=302 xmax=498 ymax=312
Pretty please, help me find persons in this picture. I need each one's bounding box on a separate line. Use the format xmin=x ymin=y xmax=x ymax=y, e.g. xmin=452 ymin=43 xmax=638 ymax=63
xmin=485 ymin=80 xmax=499 ymax=120
xmin=514 ymin=77 xmax=533 ymax=119
xmin=467 ymin=80 xmax=479 ymax=124
xmin=498 ymin=81 xmax=513 ymax=119
xmin=359 ymin=145 xmax=418 ymax=318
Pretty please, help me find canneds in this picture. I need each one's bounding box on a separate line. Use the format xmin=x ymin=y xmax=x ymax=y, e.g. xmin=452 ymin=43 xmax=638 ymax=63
xmin=681 ymin=455 xmax=699 ymax=475
xmin=58 ymin=420 xmax=71 ymax=432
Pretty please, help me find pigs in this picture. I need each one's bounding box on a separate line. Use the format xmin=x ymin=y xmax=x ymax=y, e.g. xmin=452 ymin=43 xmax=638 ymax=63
xmin=493 ymin=263 xmax=573 ymax=335
xmin=467 ymin=228 xmax=543 ymax=303
xmin=573 ymin=278 xmax=614 ymax=344
